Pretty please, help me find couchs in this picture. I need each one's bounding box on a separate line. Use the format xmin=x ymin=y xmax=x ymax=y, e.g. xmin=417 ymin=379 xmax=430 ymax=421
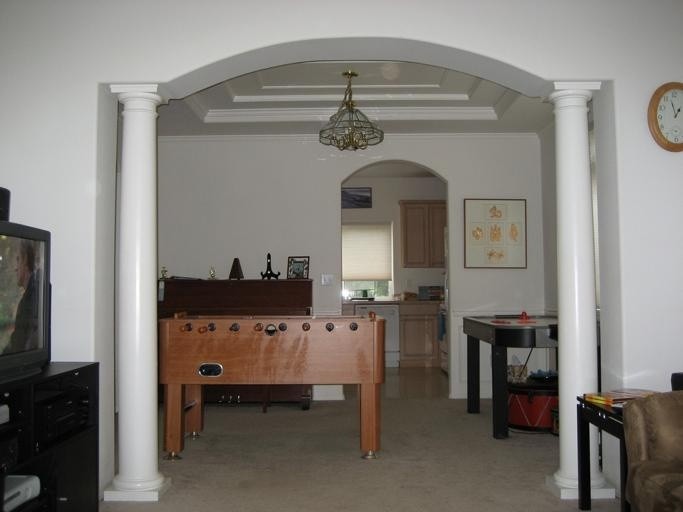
xmin=622 ymin=388 xmax=683 ymax=512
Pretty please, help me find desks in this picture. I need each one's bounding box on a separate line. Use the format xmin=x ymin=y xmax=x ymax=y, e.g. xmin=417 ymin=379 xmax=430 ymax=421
xmin=462 ymin=309 xmax=559 ymax=441
xmin=574 ymin=391 xmax=630 ymax=511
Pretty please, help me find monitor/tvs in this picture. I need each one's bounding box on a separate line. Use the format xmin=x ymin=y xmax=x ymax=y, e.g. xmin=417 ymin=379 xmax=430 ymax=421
xmin=0 ymin=221 xmax=52 ymax=384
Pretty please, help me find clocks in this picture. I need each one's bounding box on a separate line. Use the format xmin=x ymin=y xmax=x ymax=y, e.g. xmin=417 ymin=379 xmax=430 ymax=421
xmin=646 ymin=80 xmax=683 ymax=154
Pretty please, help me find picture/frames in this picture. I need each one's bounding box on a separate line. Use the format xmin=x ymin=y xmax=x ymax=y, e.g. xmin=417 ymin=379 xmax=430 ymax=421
xmin=463 ymin=197 xmax=527 ymax=269
xmin=341 ymin=186 xmax=373 ymax=209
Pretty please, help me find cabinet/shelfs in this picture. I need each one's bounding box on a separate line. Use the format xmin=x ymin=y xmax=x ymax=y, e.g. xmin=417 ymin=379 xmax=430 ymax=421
xmin=399 ymin=199 xmax=445 ymax=268
xmin=159 ymin=308 xmax=385 ymax=459
xmin=0 ymin=361 xmax=100 ymax=512
xmin=399 ymin=302 xmax=439 ymax=368
xmin=159 ymin=275 xmax=311 ymax=412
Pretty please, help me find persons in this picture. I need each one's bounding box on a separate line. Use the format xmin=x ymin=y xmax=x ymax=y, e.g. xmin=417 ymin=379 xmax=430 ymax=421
xmin=2 ymin=239 xmax=40 ymax=352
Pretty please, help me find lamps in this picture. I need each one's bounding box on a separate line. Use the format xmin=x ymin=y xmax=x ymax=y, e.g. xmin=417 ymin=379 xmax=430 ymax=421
xmin=319 ymin=70 xmax=385 ymax=152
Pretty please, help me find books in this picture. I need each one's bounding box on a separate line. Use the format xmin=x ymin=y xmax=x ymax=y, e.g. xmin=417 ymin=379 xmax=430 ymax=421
xmin=583 ymin=389 xmax=656 ymax=407
